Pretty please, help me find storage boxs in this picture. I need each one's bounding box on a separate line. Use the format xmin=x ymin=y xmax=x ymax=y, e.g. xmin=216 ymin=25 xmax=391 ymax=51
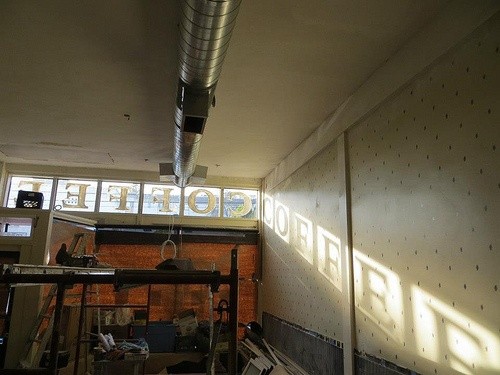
xmin=86 ymin=303 xmax=233 ymax=356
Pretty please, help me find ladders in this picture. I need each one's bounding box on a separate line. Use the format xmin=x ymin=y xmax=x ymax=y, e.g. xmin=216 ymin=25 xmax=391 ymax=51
xmin=16 ymin=232 xmax=102 ymax=375
